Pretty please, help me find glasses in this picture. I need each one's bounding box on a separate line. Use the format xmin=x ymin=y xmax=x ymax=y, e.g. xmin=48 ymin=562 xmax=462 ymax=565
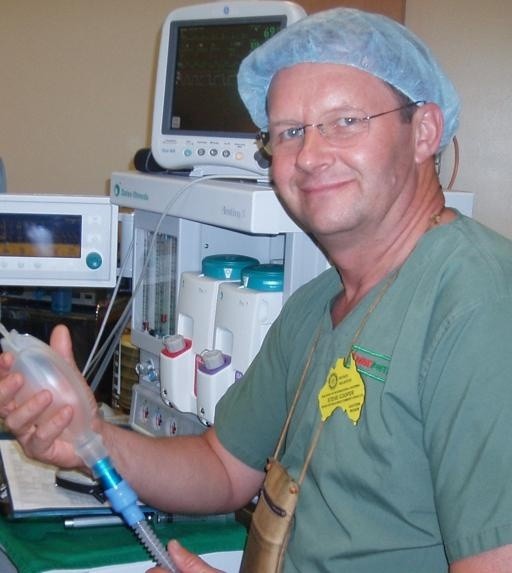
xmin=269 ymin=100 xmax=427 ymax=154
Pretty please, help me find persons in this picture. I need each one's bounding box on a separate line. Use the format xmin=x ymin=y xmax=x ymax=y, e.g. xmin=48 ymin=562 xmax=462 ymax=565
xmin=0 ymin=8 xmax=511 ymax=573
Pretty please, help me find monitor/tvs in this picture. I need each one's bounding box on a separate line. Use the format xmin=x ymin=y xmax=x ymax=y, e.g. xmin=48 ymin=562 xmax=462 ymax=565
xmin=151 ymin=1 xmax=307 ymax=183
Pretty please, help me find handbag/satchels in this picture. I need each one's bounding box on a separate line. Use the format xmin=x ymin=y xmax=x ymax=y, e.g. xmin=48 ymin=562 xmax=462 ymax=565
xmin=240 ymin=457 xmax=300 ymax=573
